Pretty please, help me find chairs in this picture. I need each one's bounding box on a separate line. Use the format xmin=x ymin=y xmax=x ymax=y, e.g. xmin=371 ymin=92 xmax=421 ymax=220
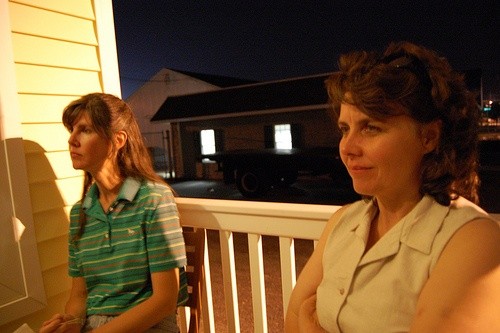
xmin=183 ymin=228 xmax=205 ymax=332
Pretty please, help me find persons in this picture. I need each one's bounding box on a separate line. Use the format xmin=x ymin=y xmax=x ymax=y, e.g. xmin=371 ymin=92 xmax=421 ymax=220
xmin=284 ymin=41 xmax=500 ymax=333
xmin=40 ymin=93 xmax=190 ymax=332
